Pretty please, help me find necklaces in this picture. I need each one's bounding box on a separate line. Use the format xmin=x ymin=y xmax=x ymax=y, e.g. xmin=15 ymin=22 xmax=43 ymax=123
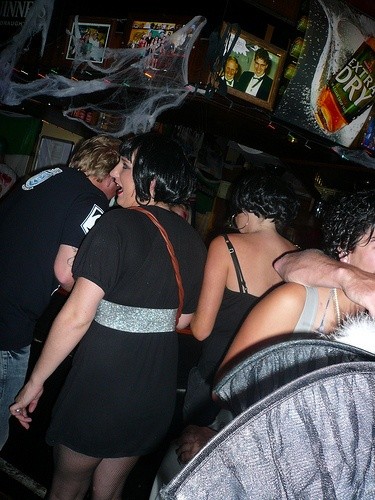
xmin=334 ymin=288 xmax=340 ymax=327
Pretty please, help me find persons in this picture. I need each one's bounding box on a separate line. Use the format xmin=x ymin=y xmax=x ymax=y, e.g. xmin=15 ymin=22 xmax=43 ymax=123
xmin=175 ymin=424 xmax=221 ymax=466
xmin=213 ymin=55 xmax=242 ymax=91
xmin=272 ymin=249 xmax=375 ymax=319
xmin=62 ymin=23 xmax=198 ymax=74
xmin=211 ymin=173 xmax=375 ymax=394
xmin=234 ymin=48 xmax=273 ymax=104
xmin=0 ymin=134 xmax=124 ymax=458
xmin=180 ymin=163 xmax=301 ymax=433
xmin=8 ymin=131 xmax=208 ymax=500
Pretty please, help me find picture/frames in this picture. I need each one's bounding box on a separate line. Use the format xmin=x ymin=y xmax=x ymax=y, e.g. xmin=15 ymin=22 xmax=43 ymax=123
xmin=216 ymin=21 xmax=288 ymax=113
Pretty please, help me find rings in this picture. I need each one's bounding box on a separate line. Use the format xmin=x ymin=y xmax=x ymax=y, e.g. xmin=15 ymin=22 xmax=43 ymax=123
xmin=16 ymin=409 xmax=22 ymax=412
xmin=19 ymin=409 xmax=22 ymax=415
